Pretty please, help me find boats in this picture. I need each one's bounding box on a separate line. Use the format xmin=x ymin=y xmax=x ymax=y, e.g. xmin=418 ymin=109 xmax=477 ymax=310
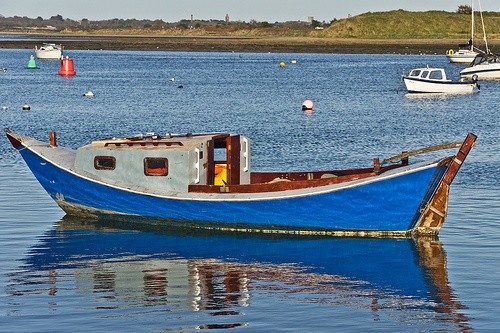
xmin=35 ymin=43 xmax=63 ymax=59
xmin=27 ymin=215 xmax=455 ymax=312
xmin=4 ymin=127 xmax=477 ymax=240
xmin=459 ymin=52 xmax=500 ymax=81
xmin=446 ymin=11 xmax=494 ymax=63
xmin=402 ymin=65 xmax=475 ymax=95
